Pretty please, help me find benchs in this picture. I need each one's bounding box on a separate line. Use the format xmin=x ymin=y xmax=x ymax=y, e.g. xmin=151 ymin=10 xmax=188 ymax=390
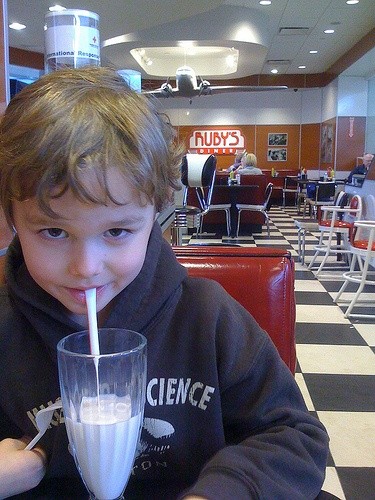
xmin=172 ymin=247 xmax=295 ymax=375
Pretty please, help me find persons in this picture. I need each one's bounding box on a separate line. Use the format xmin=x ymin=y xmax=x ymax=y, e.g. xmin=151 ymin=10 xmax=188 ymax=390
xmin=347 ymin=154 xmax=374 ymax=184
xmin=226 ymin=151 xmax=263 ymax=175
xmin=0 ymin=65 xmax=330 ymax=500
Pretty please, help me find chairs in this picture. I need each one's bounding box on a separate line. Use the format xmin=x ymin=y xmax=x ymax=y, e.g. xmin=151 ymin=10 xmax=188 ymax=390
xmin=168 ymin=155 xmax=273 ymax=246
xmin=282 ymin=175 xmax=375 ymax=318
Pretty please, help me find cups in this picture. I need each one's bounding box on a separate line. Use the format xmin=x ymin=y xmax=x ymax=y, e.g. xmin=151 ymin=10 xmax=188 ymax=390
xmin=56 ymin=327 xmax=148 ymax=500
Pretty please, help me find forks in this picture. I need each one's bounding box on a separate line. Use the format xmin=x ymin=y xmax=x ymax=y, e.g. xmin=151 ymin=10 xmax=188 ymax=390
xmin=24 ymin=400 xmax=63 ymax=451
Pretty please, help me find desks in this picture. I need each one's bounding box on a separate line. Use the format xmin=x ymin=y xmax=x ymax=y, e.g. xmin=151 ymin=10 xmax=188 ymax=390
xmin=209 ymin=185 xmax=260 ymax=238
